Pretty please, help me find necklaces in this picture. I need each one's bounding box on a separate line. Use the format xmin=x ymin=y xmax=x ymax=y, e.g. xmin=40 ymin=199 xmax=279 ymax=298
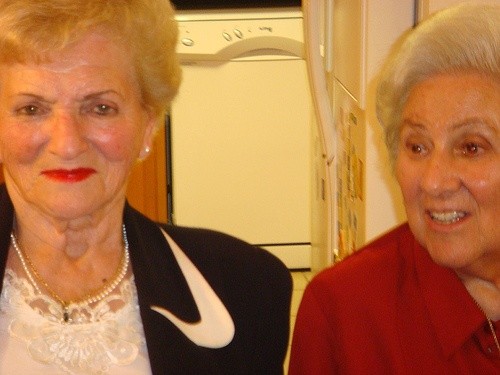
xmin=10 ymin=224 xmax=129 ymax=324
xmin=473 ymin=298 xmax=500 ymax=351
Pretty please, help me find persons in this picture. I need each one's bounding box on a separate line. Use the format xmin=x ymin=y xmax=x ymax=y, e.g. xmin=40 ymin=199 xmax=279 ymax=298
xmin=0 ymin=0 xmax=294 ymax=375
xmin=288 ymin=0 xmax=500 ymax=375
